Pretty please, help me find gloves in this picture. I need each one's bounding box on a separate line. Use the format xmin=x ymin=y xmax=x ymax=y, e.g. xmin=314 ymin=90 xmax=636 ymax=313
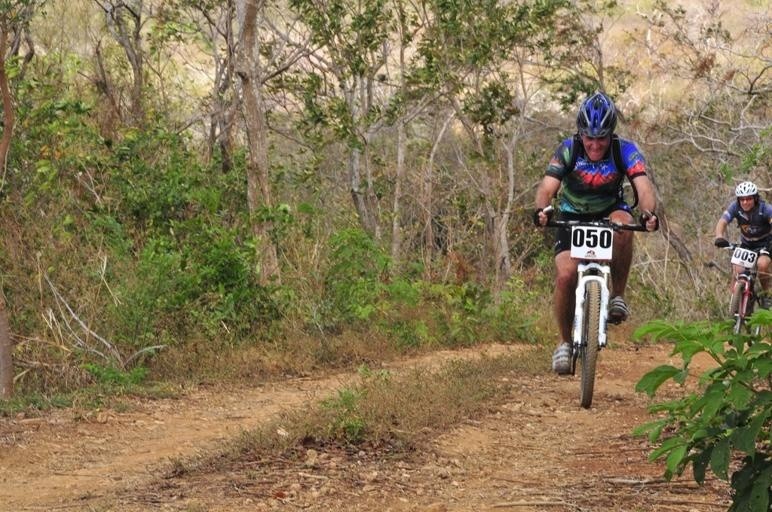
xmin=714 ymin=238 xmax=729 ymax=248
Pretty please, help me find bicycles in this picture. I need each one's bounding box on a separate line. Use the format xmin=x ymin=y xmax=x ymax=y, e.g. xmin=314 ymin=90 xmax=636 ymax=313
xmin=705 ymin=238 xmax=768 ymax=341
xmin=542 ymin=204 xmax=654 ymax=411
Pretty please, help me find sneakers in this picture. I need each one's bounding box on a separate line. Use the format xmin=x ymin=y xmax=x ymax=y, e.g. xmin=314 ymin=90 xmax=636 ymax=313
xmin=609 ymin=295 xmax=629 ymax=322
xmin=550 ymin=342 xmax=576 ymax=373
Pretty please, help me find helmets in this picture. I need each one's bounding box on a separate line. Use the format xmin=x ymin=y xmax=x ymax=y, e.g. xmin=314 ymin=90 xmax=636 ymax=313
xmin=735 ymin=180 xmax=757 ymax=198
xmin=576 ymin=92 xmax=617 ymax=138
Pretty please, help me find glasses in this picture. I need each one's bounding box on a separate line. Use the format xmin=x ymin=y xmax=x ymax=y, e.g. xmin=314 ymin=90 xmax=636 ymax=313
xmin=739 ymin=195 xmax=753 ymax=201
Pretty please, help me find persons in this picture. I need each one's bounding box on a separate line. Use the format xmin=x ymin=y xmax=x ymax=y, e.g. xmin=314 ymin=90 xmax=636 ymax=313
xmin=715 ymin=181 xmax=772 ymax=309
xmin=533 ymin=91 xmax=659 ymax=374
